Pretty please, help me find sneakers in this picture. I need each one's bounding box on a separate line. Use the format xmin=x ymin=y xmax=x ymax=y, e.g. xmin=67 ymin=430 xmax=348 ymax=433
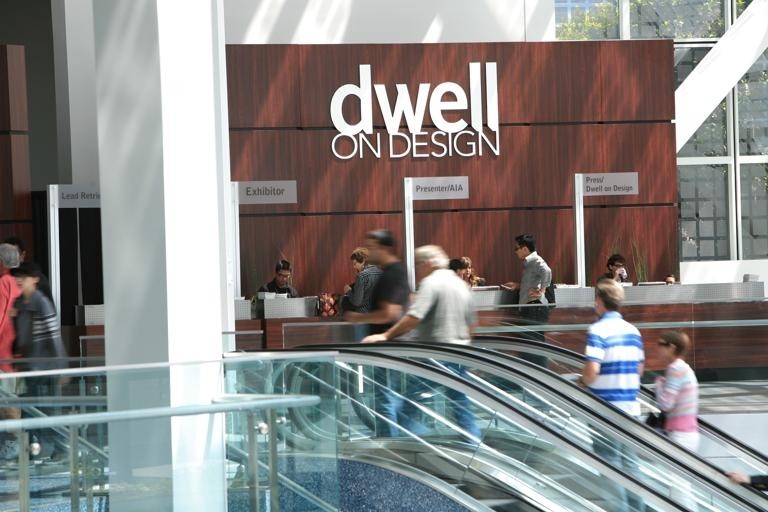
xmin=40 ymin=449 xmax=71 ymax=467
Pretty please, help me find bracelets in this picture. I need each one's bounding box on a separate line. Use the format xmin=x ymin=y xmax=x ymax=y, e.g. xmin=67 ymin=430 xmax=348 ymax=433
xmin=579 ymin=378 xmax=588 ymax=387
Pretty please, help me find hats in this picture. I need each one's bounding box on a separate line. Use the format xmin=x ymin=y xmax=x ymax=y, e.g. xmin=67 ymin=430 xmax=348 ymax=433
xmin=8 ymin=260 xmax=43 ymax=279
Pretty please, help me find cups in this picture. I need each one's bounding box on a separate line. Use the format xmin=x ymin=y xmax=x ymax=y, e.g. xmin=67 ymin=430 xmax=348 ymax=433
xmin=621 ymin=267 xmax=627 ymax=280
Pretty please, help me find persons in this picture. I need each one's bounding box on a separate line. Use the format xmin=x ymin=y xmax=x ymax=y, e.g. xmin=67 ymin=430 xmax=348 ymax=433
xmin=255 ymin=260 xmax=300 ymax=317
xmin=599 ymin=254 xmax=629 ymax=283
xmin=504 ymin=233 xmax=552 ymax=366
xmin=344 ymin=246 xmax=383 ymax=340
xmin=343 ymin=229 xmax=413 ymax=437
xmin=360 ymin=244 xmax=482 ymax=453
xmin=0 ymin=236 xmax=70 ymax=467
xmin=655 ymin=331 xmax=699 ymax=507
xmin=576 ymin=279 xmax=645 ymax=512
xmin=450 ymin=259 xmax=464 ymax=279
xmin=461 ymin=257 xmax=485 ymax=286
xmin=725 ymin=471 xmax=768 ymax=491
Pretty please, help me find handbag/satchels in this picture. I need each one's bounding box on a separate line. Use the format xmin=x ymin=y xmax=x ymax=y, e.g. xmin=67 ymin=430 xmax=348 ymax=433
xmin=644 ymin=412 xmax=668 ymax=431
